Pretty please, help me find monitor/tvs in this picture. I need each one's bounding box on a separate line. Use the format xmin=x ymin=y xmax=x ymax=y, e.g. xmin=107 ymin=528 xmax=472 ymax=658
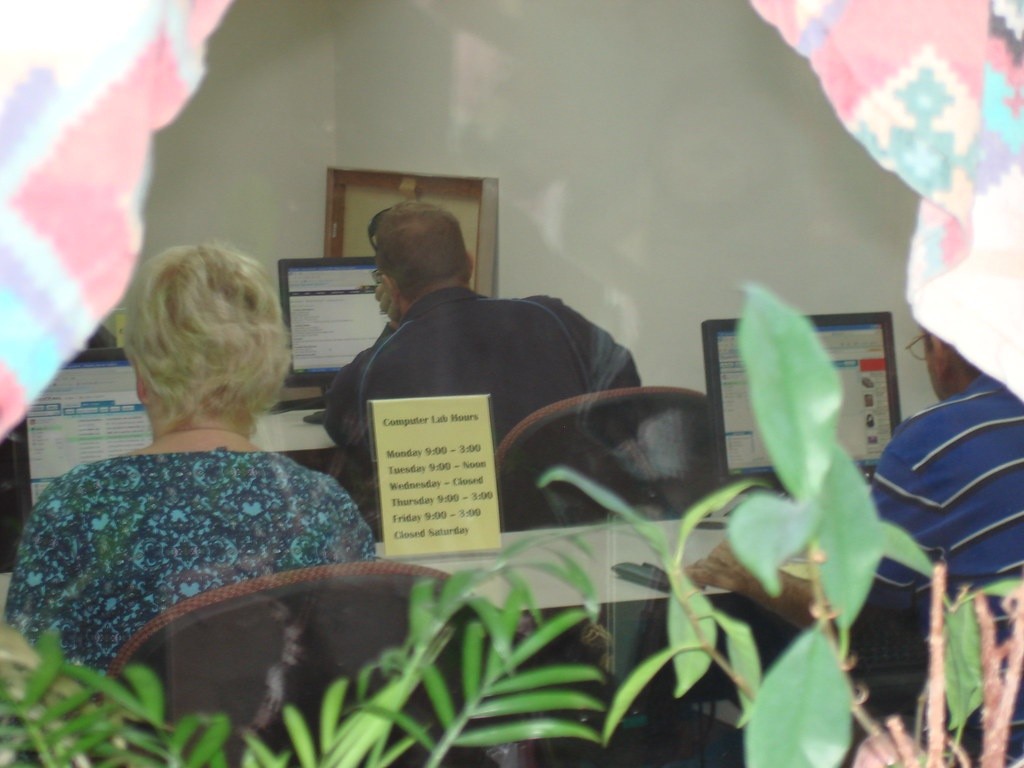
xmin=700 ymin=312 xmax=901 ymax=500
xmin=16 ymin=347 xmax=152 ymax=527
xmin=278 ymin=255 xmax=391 ymax=407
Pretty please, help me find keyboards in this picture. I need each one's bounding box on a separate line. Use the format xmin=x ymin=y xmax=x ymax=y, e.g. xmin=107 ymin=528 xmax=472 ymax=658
xmin=303 ymin=411 xmax=327 ymax=424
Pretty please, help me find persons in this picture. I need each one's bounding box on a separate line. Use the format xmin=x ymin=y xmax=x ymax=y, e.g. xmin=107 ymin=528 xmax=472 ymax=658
xmin=4 ymin=242 xmax=378 ymax=681
xmin=323 ymin=199 xmax=640 ymax=480
xmin=681 ymin=320 xmax=1024 ymax=768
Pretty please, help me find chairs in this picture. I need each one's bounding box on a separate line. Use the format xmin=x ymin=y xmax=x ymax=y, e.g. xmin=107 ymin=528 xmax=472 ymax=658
xmin=115 ymin=559 xmax=536 ymax=768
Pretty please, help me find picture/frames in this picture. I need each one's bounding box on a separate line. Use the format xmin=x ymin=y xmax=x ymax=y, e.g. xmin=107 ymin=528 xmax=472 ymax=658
xmin=326 ymin=165 xmax=501 ymax=300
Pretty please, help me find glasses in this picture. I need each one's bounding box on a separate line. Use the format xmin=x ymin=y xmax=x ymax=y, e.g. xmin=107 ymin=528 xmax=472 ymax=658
xmin=371 ymin=268 xmax=391 ymax=284
xmin=905 ymin=332 xmax=929 ymax=360
xmin=368 ymin=206 xmax=394 ymax=270
